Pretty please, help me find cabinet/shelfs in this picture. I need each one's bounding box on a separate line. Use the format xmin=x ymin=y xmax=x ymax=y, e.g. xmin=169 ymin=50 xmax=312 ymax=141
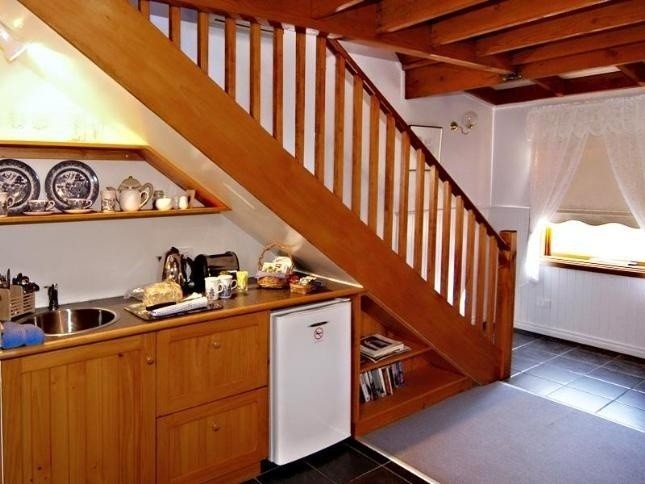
xmin=356 ymin=294 xmax=469 ymax=438
xmin=0 ymin=140 xmax=232 ymax=223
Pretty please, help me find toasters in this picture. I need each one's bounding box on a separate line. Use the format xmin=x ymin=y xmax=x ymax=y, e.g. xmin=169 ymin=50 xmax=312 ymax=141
xmin=190 ymin=250 xmax=241 ymax=292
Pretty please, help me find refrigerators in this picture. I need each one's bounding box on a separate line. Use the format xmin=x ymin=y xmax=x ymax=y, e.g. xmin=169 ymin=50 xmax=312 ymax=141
xmin=269 ymin=298 xmax=352 ymax=466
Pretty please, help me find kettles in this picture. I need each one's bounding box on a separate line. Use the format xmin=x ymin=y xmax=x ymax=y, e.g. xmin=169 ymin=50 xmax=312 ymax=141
xmin=162 ymin=247 xmax=196 ymax=292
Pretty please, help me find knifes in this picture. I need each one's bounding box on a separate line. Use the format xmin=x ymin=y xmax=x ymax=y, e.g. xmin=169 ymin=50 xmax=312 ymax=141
xmin=145 ymin=297 xmax=201 ymax=312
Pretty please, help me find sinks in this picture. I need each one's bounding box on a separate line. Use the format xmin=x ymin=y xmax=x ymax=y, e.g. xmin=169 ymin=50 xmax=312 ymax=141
xmin=17 ymin=308 xmax=117 ymax=338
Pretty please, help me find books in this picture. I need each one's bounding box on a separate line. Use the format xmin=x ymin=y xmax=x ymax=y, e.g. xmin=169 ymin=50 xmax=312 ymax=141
xmin=357 ymin=333 xmax=414 ymax=405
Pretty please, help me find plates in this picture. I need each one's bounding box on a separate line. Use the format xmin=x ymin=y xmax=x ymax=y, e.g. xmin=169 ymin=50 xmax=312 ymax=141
xmin=44 ymin=159 xmax=99 ymax=212
xmin=0 ymin=158 xmax=41 ymax=217
xmin=63 ymin=209 xmax=92 ymax=214
xmin=23 ymin=211 xmax=54 ymax=216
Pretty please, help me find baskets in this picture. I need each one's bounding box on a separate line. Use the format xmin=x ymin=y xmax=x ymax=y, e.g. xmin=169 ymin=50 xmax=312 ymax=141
xmin=257 ymin=241 xmax=294 ymax=289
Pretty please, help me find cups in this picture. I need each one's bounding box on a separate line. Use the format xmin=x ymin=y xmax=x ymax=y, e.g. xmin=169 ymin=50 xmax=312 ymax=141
xmin=100 ymin=190 xmax=116 ymax=213
xmin=27 ymin=199 xmax=55 ymax=212
xmin=0 ymin=191 xmax=15 ymax=217
xmin=66 ymin=198 xmax=92 ymax=210
xmin=217 ymin=274 xmax=237 ymax=299
xmin=204 ymin=276 xmax=223 ymax=301
xmin=236 ymin=271 xmax=249 ymax=291
xmin=155 ymin=198 xmax=173 ymax=211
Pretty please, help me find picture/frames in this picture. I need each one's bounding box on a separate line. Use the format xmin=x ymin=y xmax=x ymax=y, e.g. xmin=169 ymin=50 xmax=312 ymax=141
xmin=407 ymin=125 xmax=442 ymax=171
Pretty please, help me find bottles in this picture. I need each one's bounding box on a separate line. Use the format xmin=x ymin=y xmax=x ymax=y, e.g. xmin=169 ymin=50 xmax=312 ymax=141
xmin=154 ymin=189 xmax=164 ymax=210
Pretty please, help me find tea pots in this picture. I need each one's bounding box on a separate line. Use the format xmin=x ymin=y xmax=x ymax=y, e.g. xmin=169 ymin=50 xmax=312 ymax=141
xmin=105 ymin=175 xmax=153 ymax=212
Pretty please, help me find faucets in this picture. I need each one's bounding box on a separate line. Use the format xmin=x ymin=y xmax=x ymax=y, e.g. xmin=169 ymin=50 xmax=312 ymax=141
xmin=47 ymin=283 xmax=59 ymax=310
